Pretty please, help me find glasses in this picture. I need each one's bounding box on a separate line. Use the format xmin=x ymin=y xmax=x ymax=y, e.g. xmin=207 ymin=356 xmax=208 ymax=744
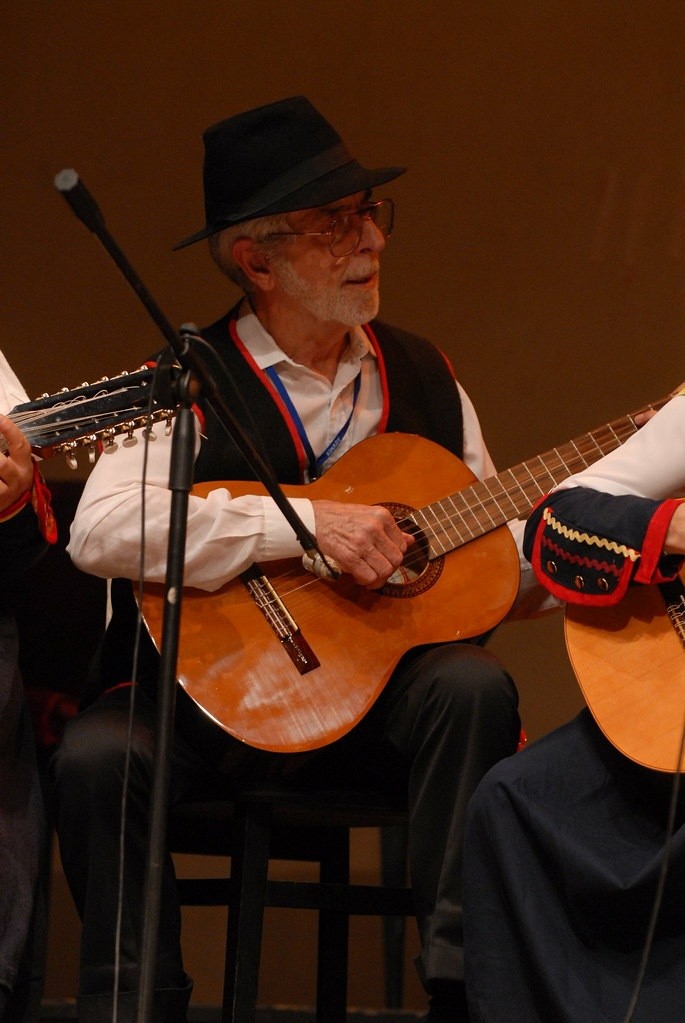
xmin=256 ymin=191 xmax=396 ymax=258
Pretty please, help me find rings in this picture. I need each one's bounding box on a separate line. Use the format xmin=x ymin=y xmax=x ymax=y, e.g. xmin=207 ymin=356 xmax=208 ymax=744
xmin=361 ymin=543 xmax=376 ymax=558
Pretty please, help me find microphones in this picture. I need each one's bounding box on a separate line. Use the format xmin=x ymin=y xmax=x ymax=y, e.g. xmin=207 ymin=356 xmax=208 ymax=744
xmin=302 ymin=551 xmax=342 ymax=581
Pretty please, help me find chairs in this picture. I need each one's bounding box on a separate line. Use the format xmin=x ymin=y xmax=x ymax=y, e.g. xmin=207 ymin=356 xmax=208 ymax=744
xmin=167 ymin=773 xmax=418 ymax=1023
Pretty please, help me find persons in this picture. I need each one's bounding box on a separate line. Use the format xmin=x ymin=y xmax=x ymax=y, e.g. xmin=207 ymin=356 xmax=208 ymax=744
xmin=0 ymin=348 xmax=92 ymax=1022
xmin=416 ymin=372 xmax=685 ymax=1023
xmin=64 ymin=94 xmax=568 ymax=1023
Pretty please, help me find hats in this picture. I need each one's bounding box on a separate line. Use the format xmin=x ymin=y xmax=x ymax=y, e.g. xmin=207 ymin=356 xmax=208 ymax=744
xmin=171 ymin=97 xmax=407 ymax=250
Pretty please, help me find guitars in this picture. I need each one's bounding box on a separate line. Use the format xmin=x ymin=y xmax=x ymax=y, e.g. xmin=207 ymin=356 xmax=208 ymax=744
xmin=0 ymin=359 xmax=198 ymax=467
xmin=132 ymin=381 xmax=685 ymax=753
xmin=563 ymin=548 xmax=685 ymax=774
